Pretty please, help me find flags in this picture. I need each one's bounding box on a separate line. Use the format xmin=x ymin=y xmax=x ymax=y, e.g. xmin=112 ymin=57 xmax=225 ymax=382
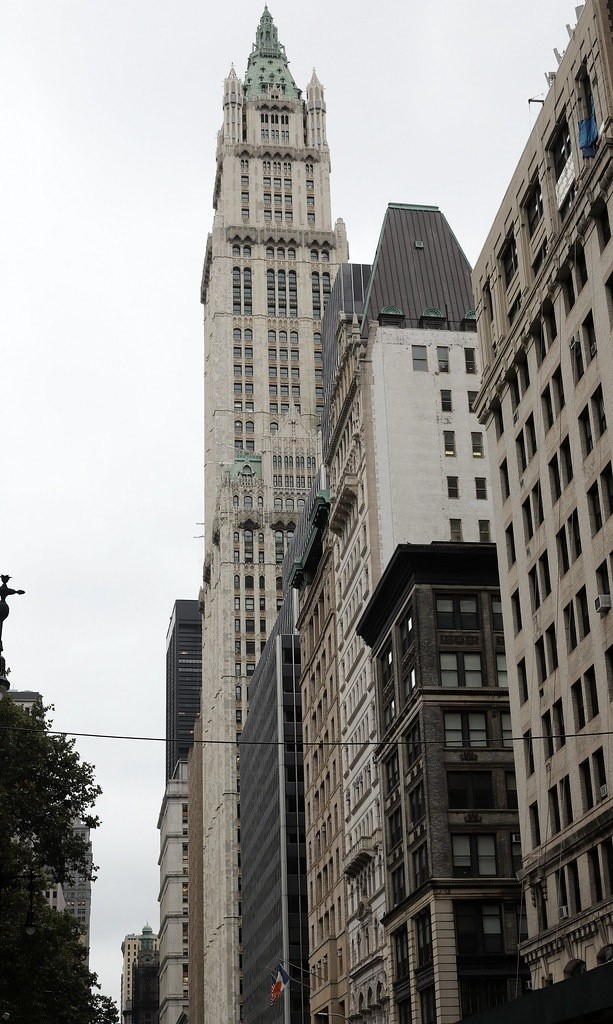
xmin=270 ymin=976 xmax=277 ymax=1006
xmin=272 ymin=963 xmax=290 ymax=1002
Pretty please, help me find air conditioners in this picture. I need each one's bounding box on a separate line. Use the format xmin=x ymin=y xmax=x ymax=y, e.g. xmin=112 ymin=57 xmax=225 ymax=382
xmin=600 ymin=785 xmax=607 ymax=798
xmin=512 ymin=833 xmax=521 ymax=843
xmin=595 ymin=595 xmax=611 ymax=614
xmin=526 ymin=980 xmax=532 ymax=990
xmin=569 ymin=334 xmax=581 ymax=349
xmin=591 ymin=342 xmax=597 ymax=355
xmin=558 ymin=905 xmax=569 ymax=920
